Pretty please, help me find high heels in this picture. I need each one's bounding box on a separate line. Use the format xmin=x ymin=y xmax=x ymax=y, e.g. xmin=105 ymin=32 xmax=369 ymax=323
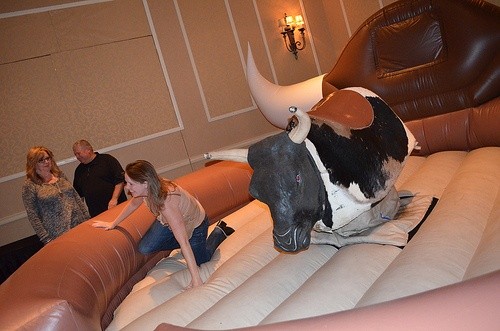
xmin=218 ymin=221 xmax=235 ymax=235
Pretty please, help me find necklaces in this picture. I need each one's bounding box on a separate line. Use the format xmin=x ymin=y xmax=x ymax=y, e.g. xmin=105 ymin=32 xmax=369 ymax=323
xmin=44 ymin=175 xmax=62 ymax=194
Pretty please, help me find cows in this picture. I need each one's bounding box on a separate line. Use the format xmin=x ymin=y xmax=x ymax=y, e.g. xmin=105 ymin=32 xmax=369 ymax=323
xmin=203 ymin=87 xmax=416 ymax=252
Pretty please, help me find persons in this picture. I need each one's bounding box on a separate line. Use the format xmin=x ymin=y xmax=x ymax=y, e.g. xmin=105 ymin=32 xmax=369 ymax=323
xmin=21 ymin=146 xmax=91 ymax=247
xmin=72 ymin=140 xmax=129 ymax=219
xmin=91 ymin=159 xmax=235 ymax=291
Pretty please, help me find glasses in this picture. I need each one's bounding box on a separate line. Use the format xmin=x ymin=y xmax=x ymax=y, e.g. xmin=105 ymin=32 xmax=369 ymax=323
xmin=39 ymin=157 xmax=52 ymax=163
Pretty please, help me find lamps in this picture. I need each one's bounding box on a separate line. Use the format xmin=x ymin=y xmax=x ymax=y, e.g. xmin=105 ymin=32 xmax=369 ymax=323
xmin=277 ymin=12 xmax=307 ymax=60
